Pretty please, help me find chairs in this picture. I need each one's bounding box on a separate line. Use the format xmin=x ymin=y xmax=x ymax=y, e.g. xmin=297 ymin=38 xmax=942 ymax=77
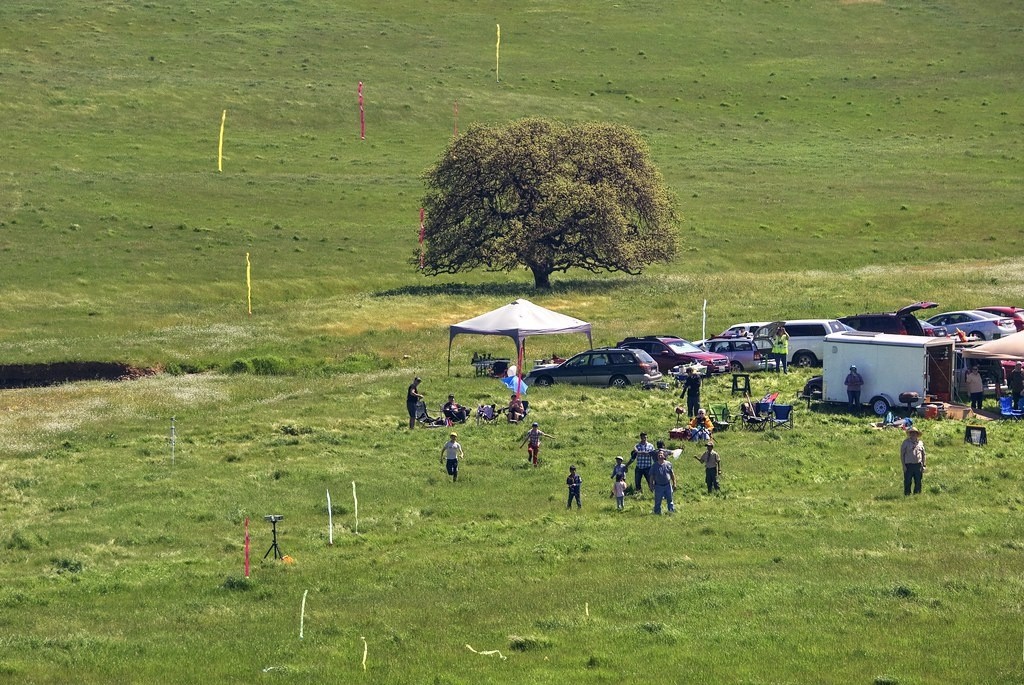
xmin=414 ymin=401 xmax=471 ymax=429
xmin=708 ymin=403 xmax=794 ymax=433
xmin=503 ymin=401 xmax=530 ymax=424
xmin=477 ymin=404 xmax=501 ymax=425
xmin=487 ymin=361 xmax=508 ymax=379
xmin=998 ymin=396 xmax=1024 ymax=423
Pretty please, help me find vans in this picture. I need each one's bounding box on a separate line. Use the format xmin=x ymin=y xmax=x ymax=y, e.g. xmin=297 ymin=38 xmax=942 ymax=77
xmin=954 ymin=350 xmax=1008 ymax=394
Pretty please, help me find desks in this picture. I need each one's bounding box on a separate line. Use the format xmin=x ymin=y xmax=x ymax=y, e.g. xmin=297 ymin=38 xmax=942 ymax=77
xmin=471 ymin=360 xmax=510 ymax=377
xmin=668 ymin=372 xmax=705 ymax=388
xmin=533 ymin=360 xmax=543 ymax=365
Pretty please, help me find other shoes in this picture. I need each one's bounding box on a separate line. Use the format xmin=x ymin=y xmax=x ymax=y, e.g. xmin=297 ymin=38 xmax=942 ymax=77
xmin=528 ymin=456 xmax=532 ymax=462
xmin=784 ymin=372 xmax=788 ymax=375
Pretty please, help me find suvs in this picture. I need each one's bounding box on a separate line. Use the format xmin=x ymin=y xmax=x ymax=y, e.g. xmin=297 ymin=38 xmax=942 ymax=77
xmin=836 ymin=301 xmax=938 ymax=336
xmin=617 ymin=335 xmax=731 ymax=378
xmin=697 ymin=321 xmax=787 ymax=372
xmin=522 ymin=346 xmax=663 ymax=389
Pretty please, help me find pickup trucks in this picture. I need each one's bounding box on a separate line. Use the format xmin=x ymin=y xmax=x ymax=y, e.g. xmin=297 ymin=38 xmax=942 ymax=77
xmin=690 ymin=319 xmax=847 ymax=368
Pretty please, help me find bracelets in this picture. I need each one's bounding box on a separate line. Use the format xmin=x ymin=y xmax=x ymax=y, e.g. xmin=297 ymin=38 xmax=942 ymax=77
xmin=718 ymin=470 xmax=721 ymax=471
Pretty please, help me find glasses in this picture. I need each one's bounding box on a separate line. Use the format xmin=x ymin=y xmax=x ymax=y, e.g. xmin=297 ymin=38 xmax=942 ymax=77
xmin=973 ymin=369 xmax=977 ymax=371
xmin=699 ymin=413 xmax=704 ymax=415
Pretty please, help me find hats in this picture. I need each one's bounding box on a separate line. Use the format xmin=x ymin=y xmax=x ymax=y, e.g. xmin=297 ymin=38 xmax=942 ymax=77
xmin=448 ymin=394 xmax=454 ymax=398
xmin=511 ymin=395 xmax=516 ymax=398
xmin=906 ymin=427 xmax=922 ymax=438
xmin=615 ymin=456 xmax=623 ymax=461
xmin=849 ymin=365 xmax=856 ymax=370
xmin=532 ymin=422 xmax=538 ymax=427
xmin=705 ymin=442 xmax=714 ymax=447
xmin=1016 ymin=361 xmax=1022 ymax=366
xmin=640 ymin=432 xmax=646 ymax=436
xmin=450 ymin=432 xmax=457 ymax=436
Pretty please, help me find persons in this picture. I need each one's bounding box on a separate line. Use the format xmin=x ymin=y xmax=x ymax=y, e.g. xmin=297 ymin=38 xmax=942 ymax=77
xmin=900 ymin=427 xmax=926 ymax=494
xmin=1009 ymin=361 xmax=1024 ymax=409
xmin=627 ymin=432 xmax=683 ymax=514
xmin=967 ymin=366 xmax=984 ymax=411
xmin=442 ymin=395 xmax=470 ymax=424
xmin=680 ymin=367 xmax=714 ymax=441
xmin=407 ymin=377 xmax=423 ymax=429
xmin=507 ymin=395 xmax=524 ymax=424
xmin=844 ymin=365 xmax=864 ymax=413
xmin=566 ymin=465 xmax=582 ymax=508
xmin=883 ymin=411 xmax=912 ymax=427
xmin=739 ymin=327 xmax=748 ymax=336
xmin=440 ymin=432 xmax=463 ymax=482
xmin=771 ymin=327 xmax=789 ymax=374
xmin=609 ymin=456 xmax=628 ymax=510
xmin=519 ymin=422 xmax=556 ymax=466
xmin=693 ymin=441 xmax=722 ymax=493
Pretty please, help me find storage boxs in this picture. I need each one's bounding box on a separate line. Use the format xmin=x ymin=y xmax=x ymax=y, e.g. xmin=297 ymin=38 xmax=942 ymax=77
xmin=916 ymin=404 xmax=944 ymax=418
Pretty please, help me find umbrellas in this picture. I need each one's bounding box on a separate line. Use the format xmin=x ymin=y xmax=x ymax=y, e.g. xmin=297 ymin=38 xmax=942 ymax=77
xmin=502 ymin=375 xmax=528 ymax=396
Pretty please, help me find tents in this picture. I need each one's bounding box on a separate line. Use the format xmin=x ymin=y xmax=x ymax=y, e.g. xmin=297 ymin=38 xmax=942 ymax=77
xmin=447 ymin=298 xmax=592 ymax=377
xmin=962 ymin=329 xmax=1024 ymax=361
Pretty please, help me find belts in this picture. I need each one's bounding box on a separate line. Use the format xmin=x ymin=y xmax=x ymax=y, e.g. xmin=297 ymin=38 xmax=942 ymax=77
xmin=657 ymin=483 xmax=669 ymax=486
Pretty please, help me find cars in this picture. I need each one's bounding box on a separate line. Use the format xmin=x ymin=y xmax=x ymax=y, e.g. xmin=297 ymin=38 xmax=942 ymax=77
xmin=924 ymin=310 xmax=1018 ymax=341
xmin=917 ymin=319 xmax=948 ymax=338
xmin=975 ymin=306 xmax=1024 ymax=333
